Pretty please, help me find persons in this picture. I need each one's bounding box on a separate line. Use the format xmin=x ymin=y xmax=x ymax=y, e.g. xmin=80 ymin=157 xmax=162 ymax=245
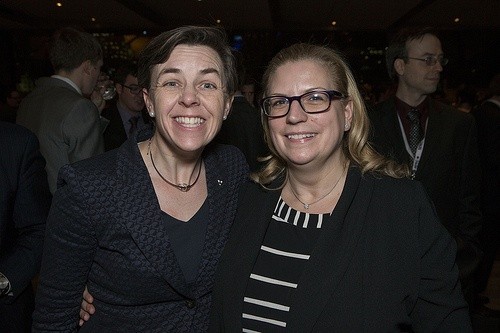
xmin=78 ymin=41 xmax=474 ymax=333
xmin=364 ymin=26 xmax=482 ymax=298
xmin=0 ymin=26 xmax=500 ymax=311
xmin=1 ymin=118 xmax=53 ymax=333
xmin=28 ymin=24 xmax=253 ymax=333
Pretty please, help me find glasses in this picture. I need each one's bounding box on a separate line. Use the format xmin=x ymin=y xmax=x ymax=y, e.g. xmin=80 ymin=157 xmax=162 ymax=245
xmin=261 ymin=90 xmax=347 ymax=118
xmin=122 ymin=83 xmax=143 ymax=95
xmin=399 ymin=56 xmax=449 ymax=67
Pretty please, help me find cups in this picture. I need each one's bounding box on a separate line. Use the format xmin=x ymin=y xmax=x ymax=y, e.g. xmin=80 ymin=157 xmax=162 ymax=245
xmin=95 ymin=72 xmax=117 ymax=100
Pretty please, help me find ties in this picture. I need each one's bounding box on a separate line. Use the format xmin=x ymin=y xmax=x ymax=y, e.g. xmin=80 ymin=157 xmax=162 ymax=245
xmin=128 ymin=114 xmax=141 ymax=136
xmin=407 ymin=106 xmax=422 ymax=168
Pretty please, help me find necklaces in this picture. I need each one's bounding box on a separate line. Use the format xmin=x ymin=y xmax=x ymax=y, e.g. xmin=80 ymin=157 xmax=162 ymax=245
xmin=288 ymin=158 xmax=348 ymax=209
xmin=149 ymin=137 xmax=207 ymax=193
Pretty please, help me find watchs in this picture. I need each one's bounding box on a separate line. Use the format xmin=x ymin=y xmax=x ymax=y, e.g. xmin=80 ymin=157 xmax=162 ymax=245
xmin=0 ymin=272 xmax=12 ymax=295
